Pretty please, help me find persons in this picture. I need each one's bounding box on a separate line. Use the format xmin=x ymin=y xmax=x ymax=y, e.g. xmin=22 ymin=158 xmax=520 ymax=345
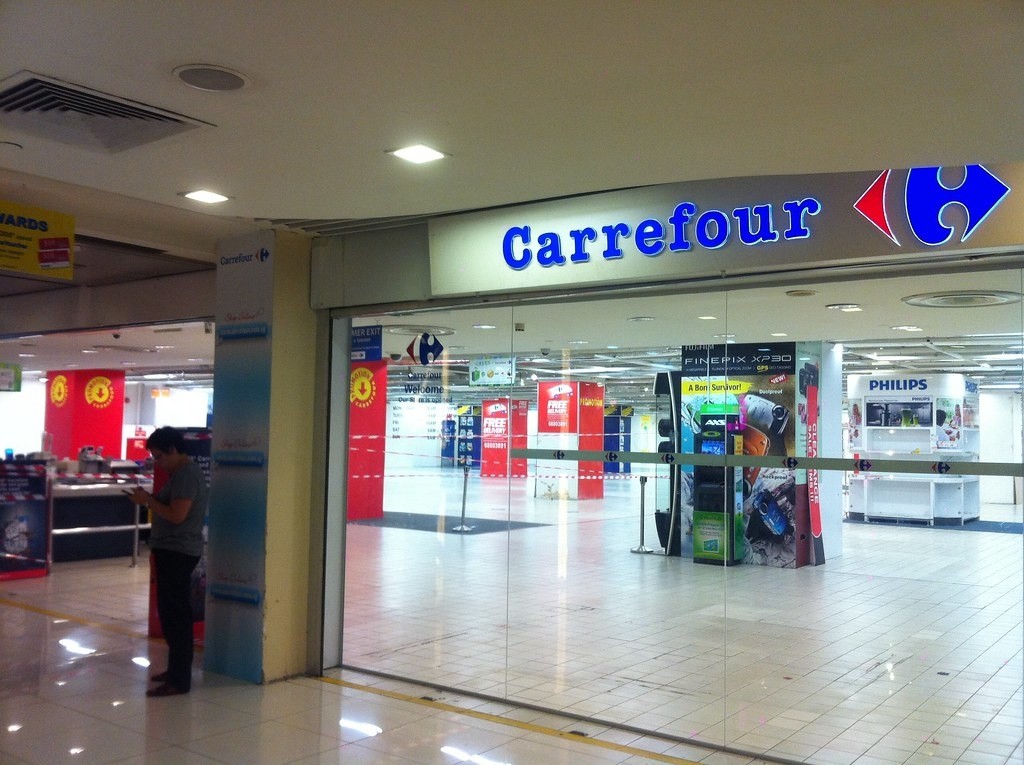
xmin=867 ymin=403 xmax=883 ymax=426
xmin=127 ymin=427 xmax=206 ymax=698
xmin=946 ymin=404 xmax=961 ymax=440
xmin=936 ymin=410 xmax=950 ymax=441
xmin=78 ymin=446 xmax=103 ymax=472
xmin=849 ymin=404 xmax=862 ymax=440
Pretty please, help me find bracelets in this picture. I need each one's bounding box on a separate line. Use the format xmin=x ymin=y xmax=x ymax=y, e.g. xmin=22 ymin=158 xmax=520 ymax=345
xmin=146 ymin=496 xmax=152 ymax=508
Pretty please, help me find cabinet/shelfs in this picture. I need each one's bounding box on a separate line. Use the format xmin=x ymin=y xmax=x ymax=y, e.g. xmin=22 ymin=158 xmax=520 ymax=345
xmin=847 ymin=394 xmax=981 ymax=527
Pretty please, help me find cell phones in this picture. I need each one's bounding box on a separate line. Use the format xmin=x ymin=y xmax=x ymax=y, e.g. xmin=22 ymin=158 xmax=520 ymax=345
xmin=122 ymin=490 xmax=133 ymax=495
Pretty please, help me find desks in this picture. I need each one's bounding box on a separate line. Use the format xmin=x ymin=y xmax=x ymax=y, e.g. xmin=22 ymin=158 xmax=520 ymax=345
xmin=45 ymin=473 xmax=153 ymax=568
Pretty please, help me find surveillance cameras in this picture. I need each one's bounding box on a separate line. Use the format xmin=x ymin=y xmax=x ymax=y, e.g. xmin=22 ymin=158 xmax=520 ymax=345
xmin=391 ymin=354 xmax=402 ymax=361
xmin=113 ymin=333 xmax=120 ymax=339
xmin=541 ymin=349 xmax=550 ymax=356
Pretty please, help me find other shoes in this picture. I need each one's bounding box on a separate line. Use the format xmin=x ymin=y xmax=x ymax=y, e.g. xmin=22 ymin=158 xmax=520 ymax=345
xmin=146 ymin=682 xmax=189 ymax=696
xmin=151 ymin=670 xmax=171 ymax=682
